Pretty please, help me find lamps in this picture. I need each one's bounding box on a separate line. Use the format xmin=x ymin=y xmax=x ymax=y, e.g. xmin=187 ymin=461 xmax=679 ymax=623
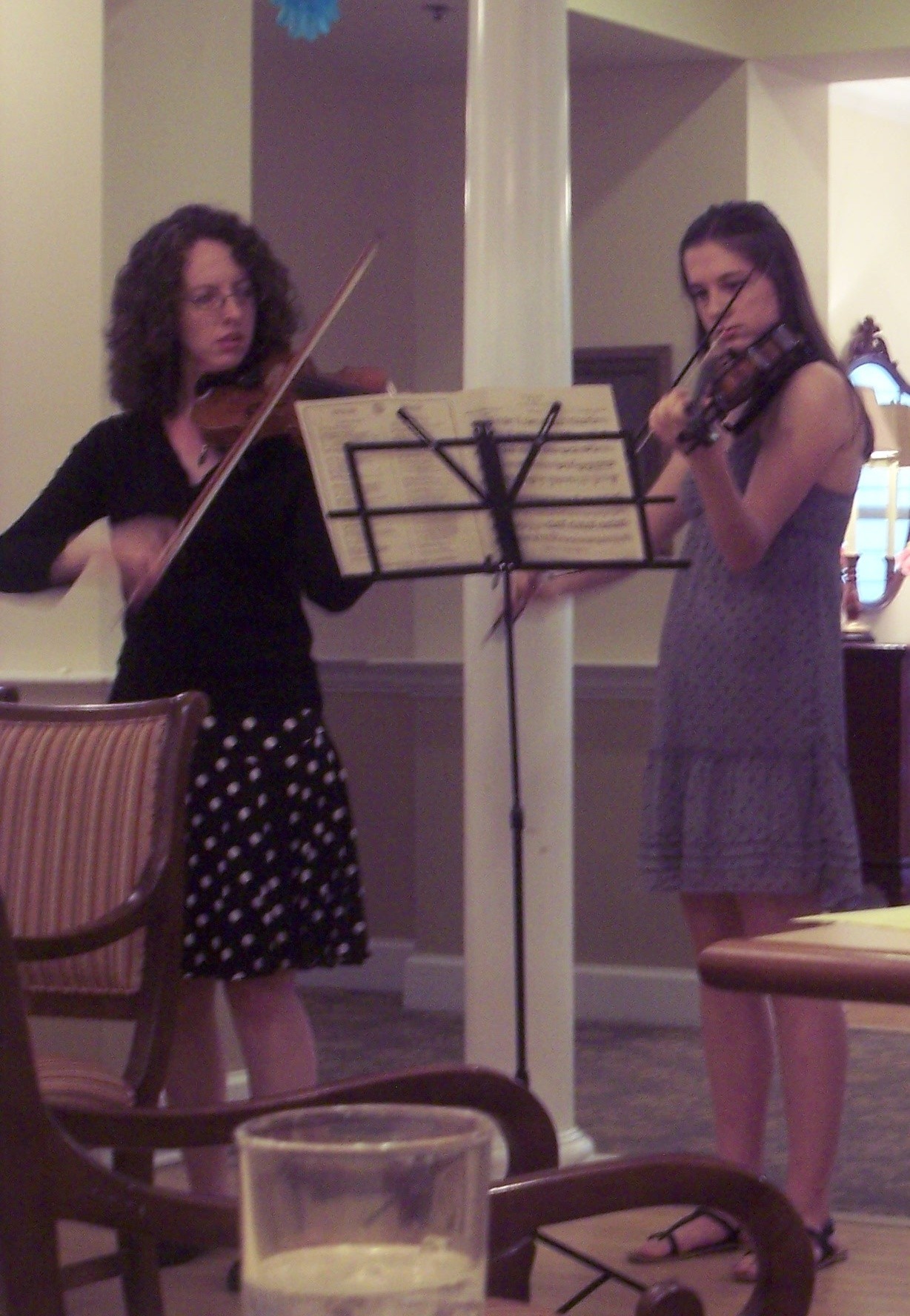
xmin=879 ymin=403 xmax=910 ymax=582
xmin=842 ymin=382 xmax=874 ymax=643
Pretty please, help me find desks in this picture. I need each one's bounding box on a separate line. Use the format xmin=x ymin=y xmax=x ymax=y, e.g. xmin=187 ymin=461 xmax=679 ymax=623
xmin=697 ymin=905 xmax=910 ymax=1010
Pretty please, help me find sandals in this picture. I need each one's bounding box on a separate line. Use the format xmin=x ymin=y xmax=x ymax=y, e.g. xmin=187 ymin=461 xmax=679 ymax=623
xmin=629 ymin=1205 xmax=754 ymax=1264
xmin=731 ymin=1216 xmax=849 ymax=1283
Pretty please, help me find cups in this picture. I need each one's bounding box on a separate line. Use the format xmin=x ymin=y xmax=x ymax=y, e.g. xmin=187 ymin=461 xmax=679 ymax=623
xmin=234 ymin=1103 xmax=494 ymax=1315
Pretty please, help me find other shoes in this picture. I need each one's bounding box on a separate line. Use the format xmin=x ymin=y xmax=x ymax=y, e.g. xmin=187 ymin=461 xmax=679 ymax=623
xmin=228 ymin=1259 xmax=243 ymax=1289
xmin=158 ymin=1238 xmax=211 ymax=1266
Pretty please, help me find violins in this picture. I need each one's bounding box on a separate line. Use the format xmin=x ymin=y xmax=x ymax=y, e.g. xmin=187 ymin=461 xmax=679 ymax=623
xmin=675 ymin=321 xmax=801 ymax=456
xmin=190 ymin=348 xmax=398 ymax=450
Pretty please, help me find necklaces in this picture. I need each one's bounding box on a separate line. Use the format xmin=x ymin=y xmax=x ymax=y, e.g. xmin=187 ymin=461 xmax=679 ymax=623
xmin=161 ymin=402 xmax=224 ymax=474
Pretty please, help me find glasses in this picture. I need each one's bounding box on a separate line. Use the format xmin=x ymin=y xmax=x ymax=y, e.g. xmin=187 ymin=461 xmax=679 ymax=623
xmin=178 ymin=278 xmax=266 ymax=313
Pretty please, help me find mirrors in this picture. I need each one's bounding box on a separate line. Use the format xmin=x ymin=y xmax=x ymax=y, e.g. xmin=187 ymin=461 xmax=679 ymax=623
xmin=842 ymin=317 xmax=910 ymax=613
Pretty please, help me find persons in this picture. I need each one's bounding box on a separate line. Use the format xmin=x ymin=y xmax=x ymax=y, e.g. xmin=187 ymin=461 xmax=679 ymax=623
xmin=492 ymin=198 xmax=875 ymax=1283
xmin=0 ymin=202 xmax=394 ymax=1299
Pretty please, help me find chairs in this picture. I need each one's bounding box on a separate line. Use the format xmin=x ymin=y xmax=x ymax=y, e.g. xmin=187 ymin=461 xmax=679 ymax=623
xmin=3 ymin=920 xmax=815 ymax=1316
xmin=1 ymin=692 xmax=211 ymax=1117
